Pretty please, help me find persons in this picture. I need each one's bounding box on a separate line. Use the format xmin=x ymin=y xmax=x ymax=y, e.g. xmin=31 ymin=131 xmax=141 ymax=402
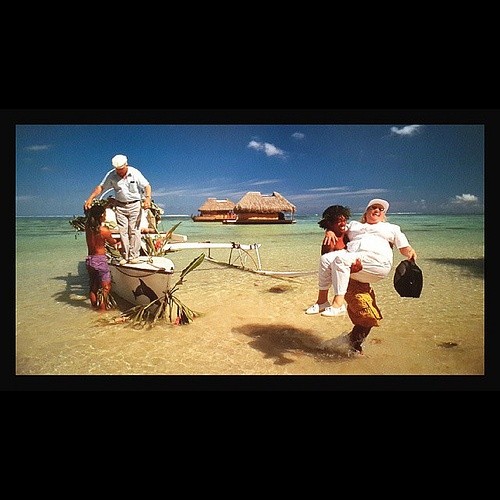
xmin=317 ymin=204 xmax=393 ymax=354
xmin=305 ymin=199 xmax=417 ymax=316
xmin=83 ymin=155 xmax=151 ymax=263
xmin=86 ymin=205 xmax=117 ymax=310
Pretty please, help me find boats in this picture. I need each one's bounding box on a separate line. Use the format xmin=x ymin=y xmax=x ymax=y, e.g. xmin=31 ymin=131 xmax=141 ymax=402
xmin=108 ymin=254 xmax=175 ymax=320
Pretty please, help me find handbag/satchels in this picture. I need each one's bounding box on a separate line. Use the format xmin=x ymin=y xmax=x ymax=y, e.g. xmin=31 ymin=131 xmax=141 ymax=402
xmin=394 ymin=256 xmax=423 ymax=298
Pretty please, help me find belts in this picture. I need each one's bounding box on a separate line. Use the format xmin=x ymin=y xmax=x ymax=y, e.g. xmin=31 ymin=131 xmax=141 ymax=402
xmin=117 ymin=200 xmax=140 ymax=204
xmin=112 ymin=155 xmax=128 ymax=170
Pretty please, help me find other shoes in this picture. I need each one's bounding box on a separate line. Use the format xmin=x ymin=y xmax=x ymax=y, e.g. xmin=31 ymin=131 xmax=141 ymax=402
xmin=321 ymin=305 xmax=347 ymax=317
xmin=131 ymin=258 xmax=140 ymax=264
xmin=305 ymin=301 xmax=331 ymax=315
xmin=119 ymin=258 xmax=129 ymax=265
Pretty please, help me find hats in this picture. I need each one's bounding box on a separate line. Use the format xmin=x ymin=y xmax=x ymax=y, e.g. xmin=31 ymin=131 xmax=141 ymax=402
xmin=365 ymin=199 xmax=390 ymax=212
